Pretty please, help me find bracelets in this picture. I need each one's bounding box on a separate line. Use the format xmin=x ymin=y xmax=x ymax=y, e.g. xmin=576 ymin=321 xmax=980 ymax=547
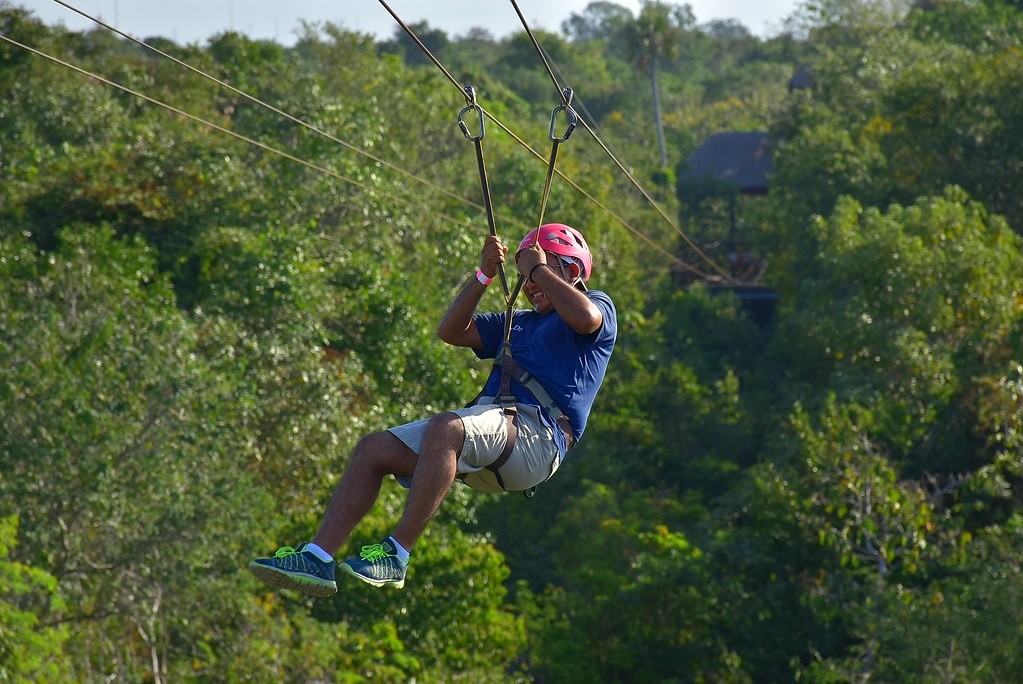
xmin=529 ymin=263 xmax=553 ymax=284
xmin=474 ymin=269 xmax=491 ymax=286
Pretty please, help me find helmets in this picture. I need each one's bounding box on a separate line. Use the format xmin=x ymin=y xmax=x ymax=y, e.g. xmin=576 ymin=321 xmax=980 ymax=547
xmin=515 ymin=223 xmax=593 ymax=282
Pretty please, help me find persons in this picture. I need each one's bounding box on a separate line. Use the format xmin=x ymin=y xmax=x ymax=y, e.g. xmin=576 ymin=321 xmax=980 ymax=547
xmin=247 ymin=223 xmax=617 ymax=596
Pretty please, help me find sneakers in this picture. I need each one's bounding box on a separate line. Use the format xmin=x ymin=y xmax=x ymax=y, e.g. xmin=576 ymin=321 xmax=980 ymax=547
xmin=338 ymin=537 xmax=410 ymax=589
xmin=249 ymin=543 xmax=339 ymax=598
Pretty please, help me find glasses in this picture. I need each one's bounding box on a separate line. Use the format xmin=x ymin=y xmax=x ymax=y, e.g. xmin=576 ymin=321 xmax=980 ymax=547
xmin=517 ymin=263 xmax=568 ymax=285
